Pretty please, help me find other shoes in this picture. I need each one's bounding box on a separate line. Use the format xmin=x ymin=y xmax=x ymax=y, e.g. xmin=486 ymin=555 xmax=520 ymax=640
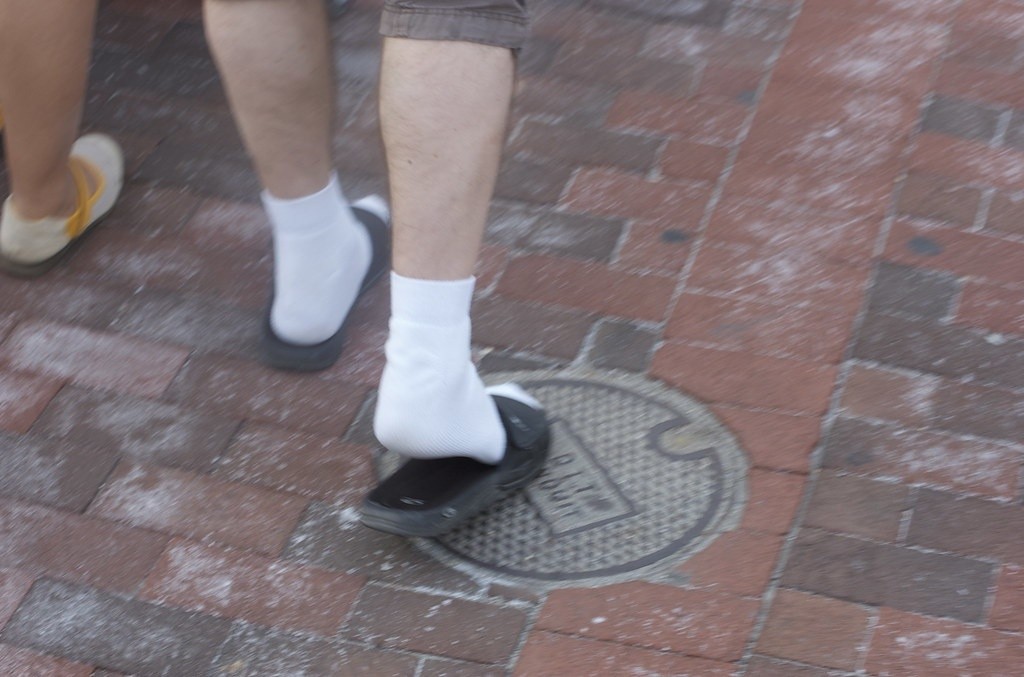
xmin=0 ymin=134 xmax=124 ymax=275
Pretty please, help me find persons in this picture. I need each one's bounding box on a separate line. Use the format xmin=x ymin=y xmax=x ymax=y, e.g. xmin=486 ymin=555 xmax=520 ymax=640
xmin=203 ymin=0 xmax=551 ymax=537
xmin=0 ymin=0 xmax=124 ymax=277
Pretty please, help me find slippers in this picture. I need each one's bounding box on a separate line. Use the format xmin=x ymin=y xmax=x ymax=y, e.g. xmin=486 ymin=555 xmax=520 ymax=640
xmin=361 ymin=393 xmax=552 ymax=534
xmin=260 ymin=198 xmax=393 ymax=373
